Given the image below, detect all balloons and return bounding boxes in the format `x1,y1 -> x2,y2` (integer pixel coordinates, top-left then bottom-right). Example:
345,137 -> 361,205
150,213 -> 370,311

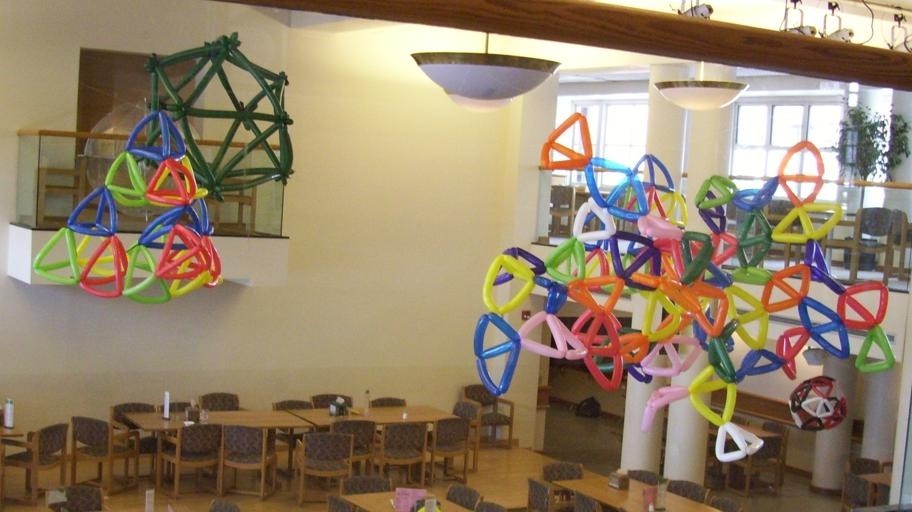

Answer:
31,32 -> 294,300
477,115 -> 897,463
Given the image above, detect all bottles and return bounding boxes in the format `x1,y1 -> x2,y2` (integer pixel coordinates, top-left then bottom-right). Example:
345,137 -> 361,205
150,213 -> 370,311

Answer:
363,389 -> 372,417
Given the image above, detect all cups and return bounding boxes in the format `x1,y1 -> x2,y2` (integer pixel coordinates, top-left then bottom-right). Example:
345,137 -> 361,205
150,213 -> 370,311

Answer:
425,497 -> 436,511
640,486 -> 666,512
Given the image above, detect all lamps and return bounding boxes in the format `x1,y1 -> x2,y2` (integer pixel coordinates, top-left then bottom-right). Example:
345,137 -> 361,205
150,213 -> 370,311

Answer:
778,0 -> 817,37
887,12 -> 912,53
820,0 -> 855,43
676,1 -> 713,19
410,30 -> 562,100
650,61 -> 749,111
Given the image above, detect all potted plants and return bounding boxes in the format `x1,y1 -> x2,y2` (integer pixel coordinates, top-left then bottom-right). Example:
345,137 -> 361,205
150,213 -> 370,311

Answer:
824,97 -> 911,271
